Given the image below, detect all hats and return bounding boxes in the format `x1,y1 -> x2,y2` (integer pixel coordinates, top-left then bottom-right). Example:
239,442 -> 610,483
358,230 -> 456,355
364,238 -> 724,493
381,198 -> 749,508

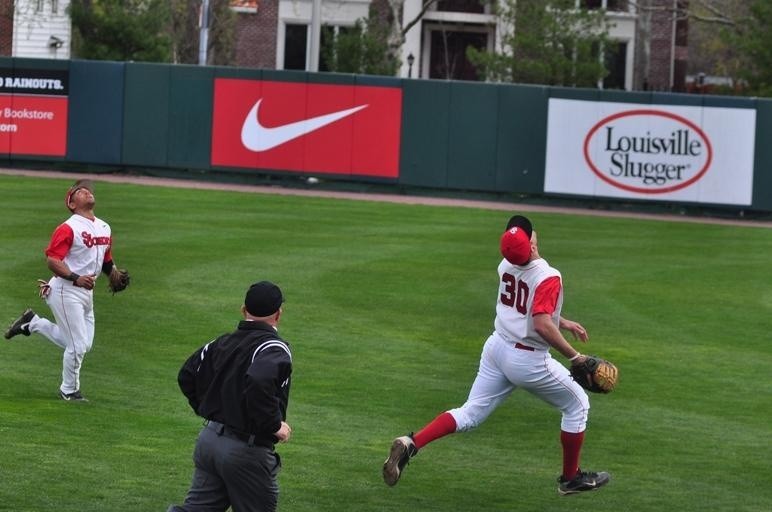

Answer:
245,280 -> 286,317
500,215 -> 532,265
65,179 -> 96,213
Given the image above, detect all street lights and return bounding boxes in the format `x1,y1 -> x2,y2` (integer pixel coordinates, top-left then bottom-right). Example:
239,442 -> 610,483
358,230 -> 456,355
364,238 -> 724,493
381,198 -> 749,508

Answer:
408,54 -> 415,78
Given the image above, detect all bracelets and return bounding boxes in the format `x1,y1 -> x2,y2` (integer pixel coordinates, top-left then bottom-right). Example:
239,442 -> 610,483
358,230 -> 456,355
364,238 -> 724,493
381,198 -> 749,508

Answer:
67,272 -> 80,281
567,352 -> 582,362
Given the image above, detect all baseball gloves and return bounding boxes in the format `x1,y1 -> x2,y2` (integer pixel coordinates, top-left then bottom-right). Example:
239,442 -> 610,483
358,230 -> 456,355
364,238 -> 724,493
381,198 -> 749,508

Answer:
110,267 -> 128,291
570,354 -> 618,393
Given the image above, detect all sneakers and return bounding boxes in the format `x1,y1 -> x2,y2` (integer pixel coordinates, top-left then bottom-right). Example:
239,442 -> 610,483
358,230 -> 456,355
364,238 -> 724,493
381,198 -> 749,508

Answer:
557,470 -> 610,495
382,435 -> 416,487
4,308 -> 35,340
59,390 -> 87,401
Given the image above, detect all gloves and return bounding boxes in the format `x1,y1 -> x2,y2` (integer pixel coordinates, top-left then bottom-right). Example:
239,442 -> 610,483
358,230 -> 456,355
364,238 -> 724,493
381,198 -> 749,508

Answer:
35,279 -> 51,300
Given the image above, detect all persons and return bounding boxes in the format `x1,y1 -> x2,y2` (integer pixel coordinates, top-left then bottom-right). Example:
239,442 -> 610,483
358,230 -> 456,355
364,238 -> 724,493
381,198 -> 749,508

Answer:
5,178 -> 130,402
383,214 -> 618,495
166,280 -> 293,511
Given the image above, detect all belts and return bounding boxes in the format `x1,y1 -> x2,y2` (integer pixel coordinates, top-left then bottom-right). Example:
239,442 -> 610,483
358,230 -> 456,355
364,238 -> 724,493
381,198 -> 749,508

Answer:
208,421 -> 274,451
